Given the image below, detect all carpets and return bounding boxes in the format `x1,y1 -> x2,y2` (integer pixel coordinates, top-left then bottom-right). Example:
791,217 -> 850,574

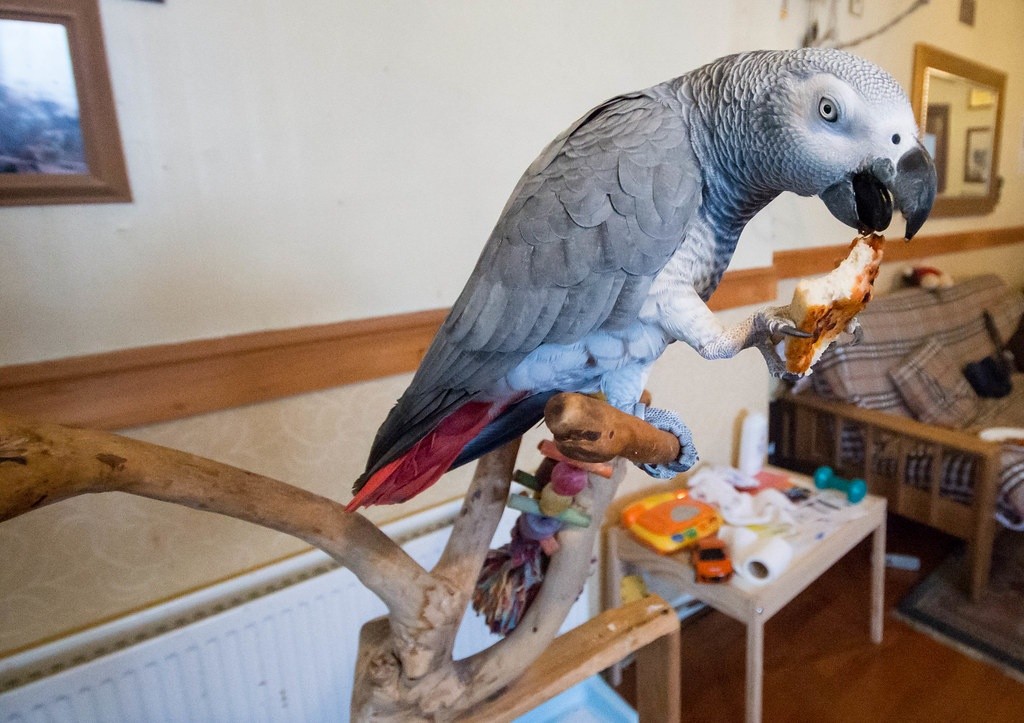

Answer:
892,536 -> 1024,684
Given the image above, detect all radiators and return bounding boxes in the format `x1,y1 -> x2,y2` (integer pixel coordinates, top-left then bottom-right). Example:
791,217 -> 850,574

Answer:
122,484 -> 590,723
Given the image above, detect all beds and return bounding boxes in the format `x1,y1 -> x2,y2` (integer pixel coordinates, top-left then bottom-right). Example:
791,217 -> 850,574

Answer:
794,392 -> 1024,604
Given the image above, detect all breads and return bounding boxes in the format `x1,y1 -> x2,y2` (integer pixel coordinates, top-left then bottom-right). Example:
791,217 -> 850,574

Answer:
785,234 -> 885,373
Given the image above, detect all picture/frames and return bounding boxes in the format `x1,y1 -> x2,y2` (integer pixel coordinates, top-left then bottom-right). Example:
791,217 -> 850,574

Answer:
926,102 -> 950,194
0,0 -> 133,205
968,87 -> 993,110
964,128 -> 992,184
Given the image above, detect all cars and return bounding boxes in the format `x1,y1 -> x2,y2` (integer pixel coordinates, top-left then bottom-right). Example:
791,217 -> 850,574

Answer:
690,538 -> 733,583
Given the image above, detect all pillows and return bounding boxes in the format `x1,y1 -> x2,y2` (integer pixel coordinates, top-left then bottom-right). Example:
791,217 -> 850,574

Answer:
889,337 -> 983,434
812,272 -> 1010,420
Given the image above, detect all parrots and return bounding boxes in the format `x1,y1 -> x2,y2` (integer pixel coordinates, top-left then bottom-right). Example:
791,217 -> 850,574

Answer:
333,48 -> 938,517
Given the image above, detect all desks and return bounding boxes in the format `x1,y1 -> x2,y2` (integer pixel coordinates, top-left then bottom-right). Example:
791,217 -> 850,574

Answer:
604,467 -> 887,723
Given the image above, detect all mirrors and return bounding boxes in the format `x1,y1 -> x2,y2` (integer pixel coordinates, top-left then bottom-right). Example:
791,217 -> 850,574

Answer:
911,43 -> 1007,219
